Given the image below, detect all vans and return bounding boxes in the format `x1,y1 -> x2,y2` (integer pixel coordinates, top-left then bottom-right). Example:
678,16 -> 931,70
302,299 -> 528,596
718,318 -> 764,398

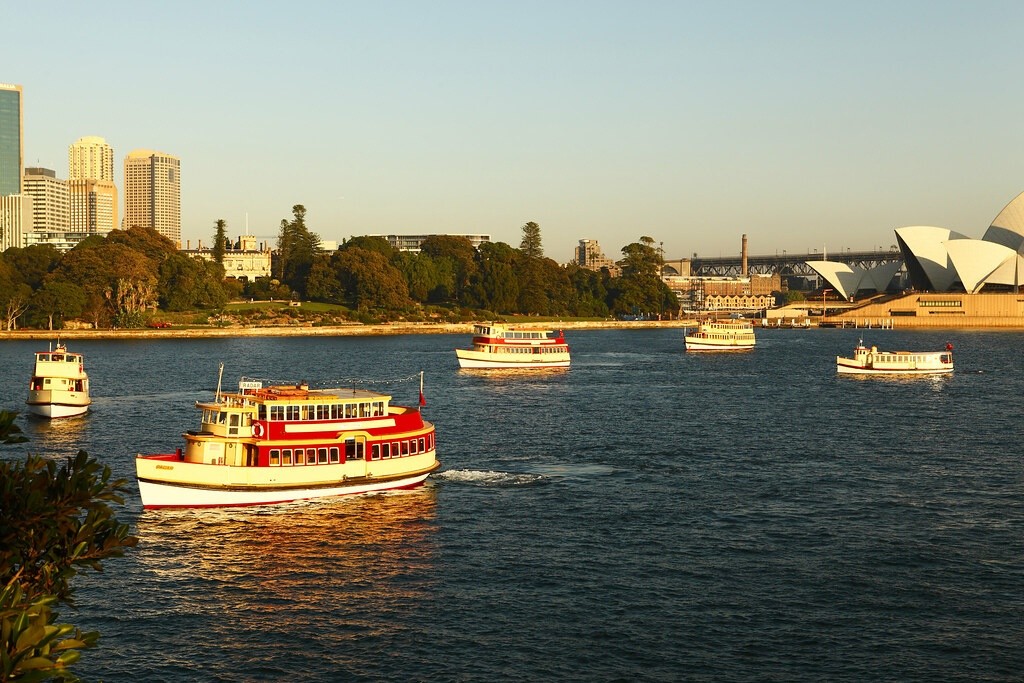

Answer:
732,313 -> 745,319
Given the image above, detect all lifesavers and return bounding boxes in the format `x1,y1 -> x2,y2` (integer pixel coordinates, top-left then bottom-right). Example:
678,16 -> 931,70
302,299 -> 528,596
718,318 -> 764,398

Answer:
251,423 -> 264,437
496,335 -> 501,340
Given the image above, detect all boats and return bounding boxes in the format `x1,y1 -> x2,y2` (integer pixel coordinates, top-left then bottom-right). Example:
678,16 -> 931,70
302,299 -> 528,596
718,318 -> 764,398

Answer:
454,317 -> 572,369
837,330 -> 955,375
136,360 -> 444,511
23,334 -> 92,424
683,305 -> 756,352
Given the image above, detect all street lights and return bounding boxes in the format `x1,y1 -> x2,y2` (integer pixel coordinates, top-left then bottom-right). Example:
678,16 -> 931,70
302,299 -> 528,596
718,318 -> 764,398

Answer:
823,289 -> 833,317
